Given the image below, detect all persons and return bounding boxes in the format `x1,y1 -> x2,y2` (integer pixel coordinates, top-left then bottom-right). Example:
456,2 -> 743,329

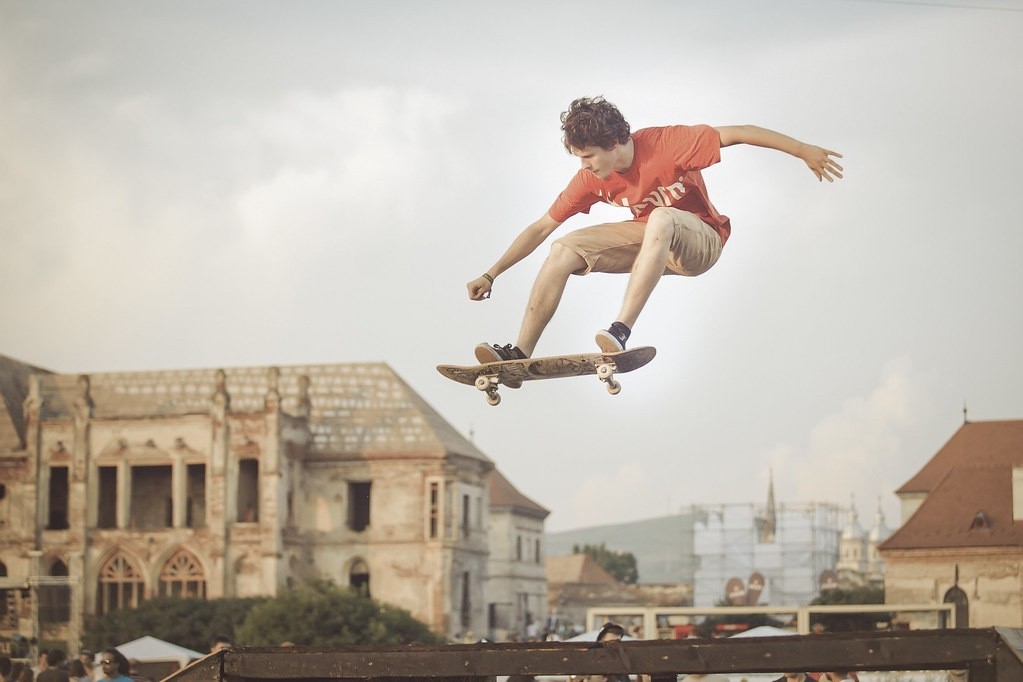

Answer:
0,657 -> 34,682
79,653 -> 96,682
467,95 -> 844,389
117,659 -> 145,682
211,637 -> 231,654
98,650 -> 135,682
31,650 -> 50,682
68,660 -> 91,682
770,671 -> 859,682
36,649 -> 69,682
573,622 -> 632,682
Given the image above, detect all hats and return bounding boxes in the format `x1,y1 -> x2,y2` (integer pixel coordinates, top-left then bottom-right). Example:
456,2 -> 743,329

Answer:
79,649 -> 95,662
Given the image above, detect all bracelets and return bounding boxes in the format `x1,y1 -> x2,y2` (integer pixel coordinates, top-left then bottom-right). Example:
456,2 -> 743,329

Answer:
483,273 -> 493,298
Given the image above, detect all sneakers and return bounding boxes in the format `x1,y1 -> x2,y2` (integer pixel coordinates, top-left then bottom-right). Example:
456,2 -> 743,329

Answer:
595,323 -> 627,353
474,342 -> 528,388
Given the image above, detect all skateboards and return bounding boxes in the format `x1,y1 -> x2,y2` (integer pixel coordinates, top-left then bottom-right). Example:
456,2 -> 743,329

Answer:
437,346 -> 658,405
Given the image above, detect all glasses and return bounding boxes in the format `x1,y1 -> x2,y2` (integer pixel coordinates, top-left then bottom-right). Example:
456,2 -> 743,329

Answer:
100,659 -> 117,664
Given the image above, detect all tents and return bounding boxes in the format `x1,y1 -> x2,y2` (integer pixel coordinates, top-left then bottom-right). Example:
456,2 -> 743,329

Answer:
95,635 -> 208,669
